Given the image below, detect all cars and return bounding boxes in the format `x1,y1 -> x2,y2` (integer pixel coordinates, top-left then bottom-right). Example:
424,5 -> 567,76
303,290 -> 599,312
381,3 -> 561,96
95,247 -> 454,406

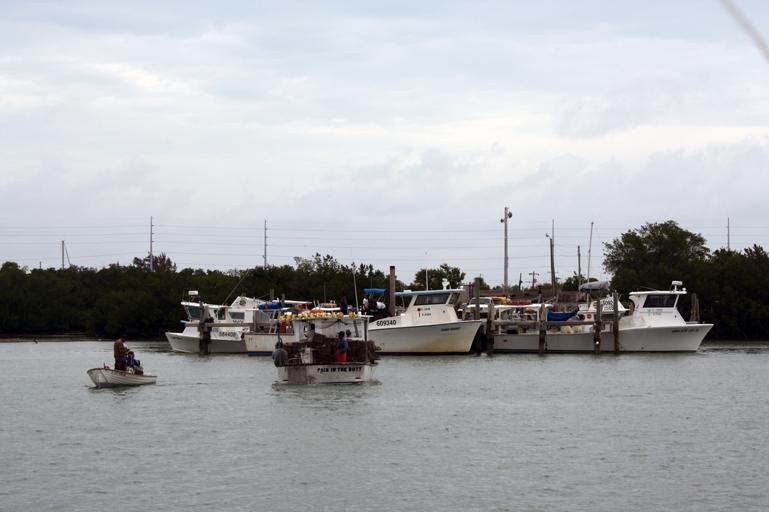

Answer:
464,296 -> 494,317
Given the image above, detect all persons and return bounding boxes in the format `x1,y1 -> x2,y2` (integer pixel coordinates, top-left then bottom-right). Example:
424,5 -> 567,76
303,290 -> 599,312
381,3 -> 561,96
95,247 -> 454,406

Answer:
376,296 -> 386,310
113,333 -> 127,372
272,341 -> 289,368
296,341 -> 320,365
304,323 -> 316,343
362,294 -> 369,315
334,330 -> 349,362
126,349 -> 145,375
340,294 -> 348,315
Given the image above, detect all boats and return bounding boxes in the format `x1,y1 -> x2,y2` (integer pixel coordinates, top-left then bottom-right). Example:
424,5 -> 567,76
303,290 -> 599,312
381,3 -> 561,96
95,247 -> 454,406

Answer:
271,305 -> 380,386
169,291 -> 310,354
85,361 -> 159,388
367,288 -> 487,355
492,283 -> 715,356
